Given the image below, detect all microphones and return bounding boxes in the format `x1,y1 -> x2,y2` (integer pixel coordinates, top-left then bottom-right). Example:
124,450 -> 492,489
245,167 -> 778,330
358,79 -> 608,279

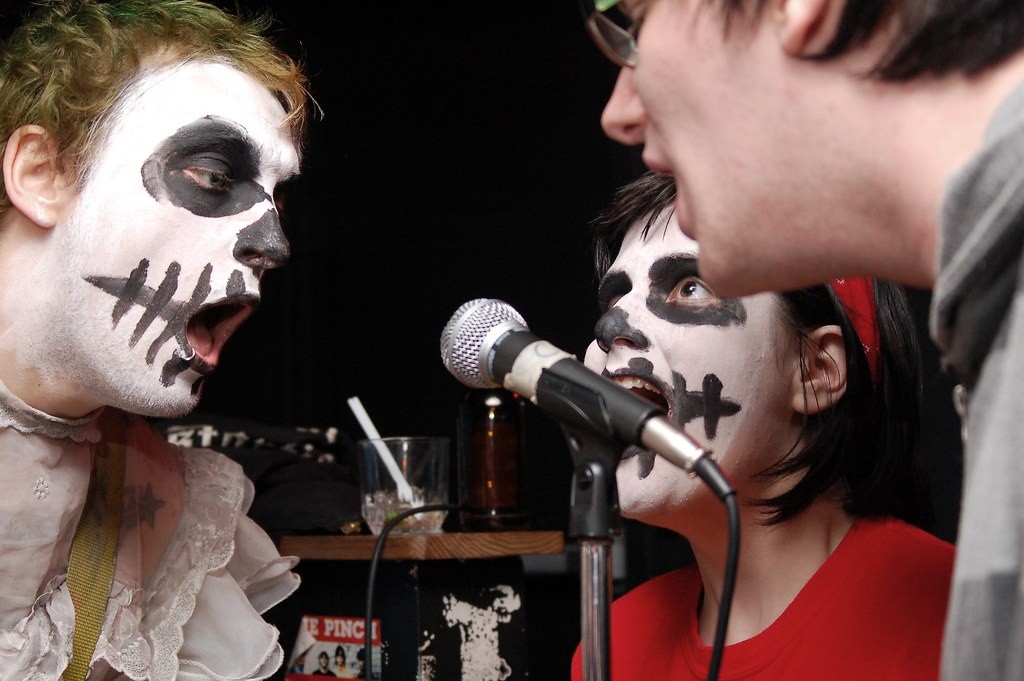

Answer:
441,298 -> 737,502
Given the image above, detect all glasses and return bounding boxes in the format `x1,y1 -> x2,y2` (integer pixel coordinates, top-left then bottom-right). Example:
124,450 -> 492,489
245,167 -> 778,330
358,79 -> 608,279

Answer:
585,0 -> 654,69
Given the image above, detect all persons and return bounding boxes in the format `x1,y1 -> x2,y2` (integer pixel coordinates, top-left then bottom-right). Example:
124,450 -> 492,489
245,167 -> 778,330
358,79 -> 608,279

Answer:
0,0 -> 326,681
571,168 -> 958,681
587,0 -> 1024,680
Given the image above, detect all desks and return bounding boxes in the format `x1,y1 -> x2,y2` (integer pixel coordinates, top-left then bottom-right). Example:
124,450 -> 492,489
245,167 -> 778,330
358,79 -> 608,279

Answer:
272,531 -> 566,681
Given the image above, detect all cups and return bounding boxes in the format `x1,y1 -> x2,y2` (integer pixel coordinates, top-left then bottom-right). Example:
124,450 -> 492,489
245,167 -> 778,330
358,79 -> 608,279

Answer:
355,435 -> 450,537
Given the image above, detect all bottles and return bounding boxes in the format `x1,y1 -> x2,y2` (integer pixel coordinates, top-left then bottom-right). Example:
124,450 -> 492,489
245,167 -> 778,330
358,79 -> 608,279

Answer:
457,384 -> 525,531
